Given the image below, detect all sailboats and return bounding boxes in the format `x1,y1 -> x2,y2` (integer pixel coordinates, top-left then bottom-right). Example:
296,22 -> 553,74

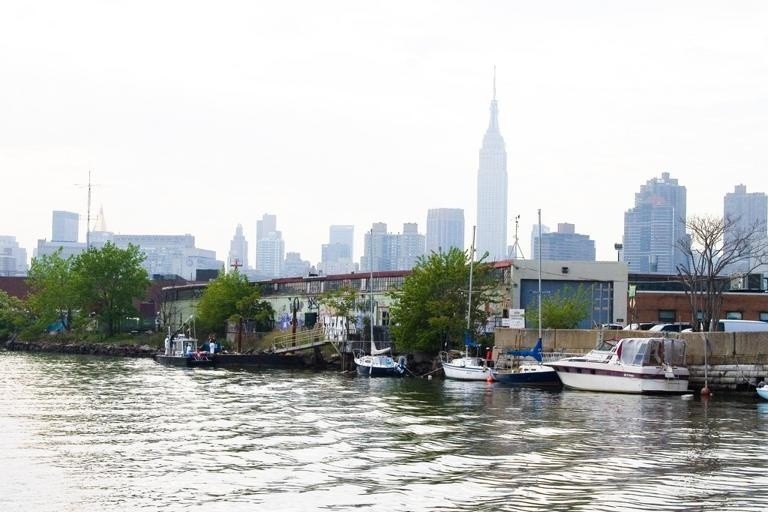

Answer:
491,207 -> 564,387
440,225 -> 506,382
353,228 -> 403,376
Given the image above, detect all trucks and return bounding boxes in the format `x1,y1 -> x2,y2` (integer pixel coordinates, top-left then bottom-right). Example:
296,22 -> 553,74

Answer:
623,317 -> 767,331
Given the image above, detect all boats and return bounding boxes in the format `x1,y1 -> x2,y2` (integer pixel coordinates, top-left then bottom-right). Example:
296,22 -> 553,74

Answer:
153,346 -> 308,371
540,337 -> 695,395
754,386 -> 768,402
